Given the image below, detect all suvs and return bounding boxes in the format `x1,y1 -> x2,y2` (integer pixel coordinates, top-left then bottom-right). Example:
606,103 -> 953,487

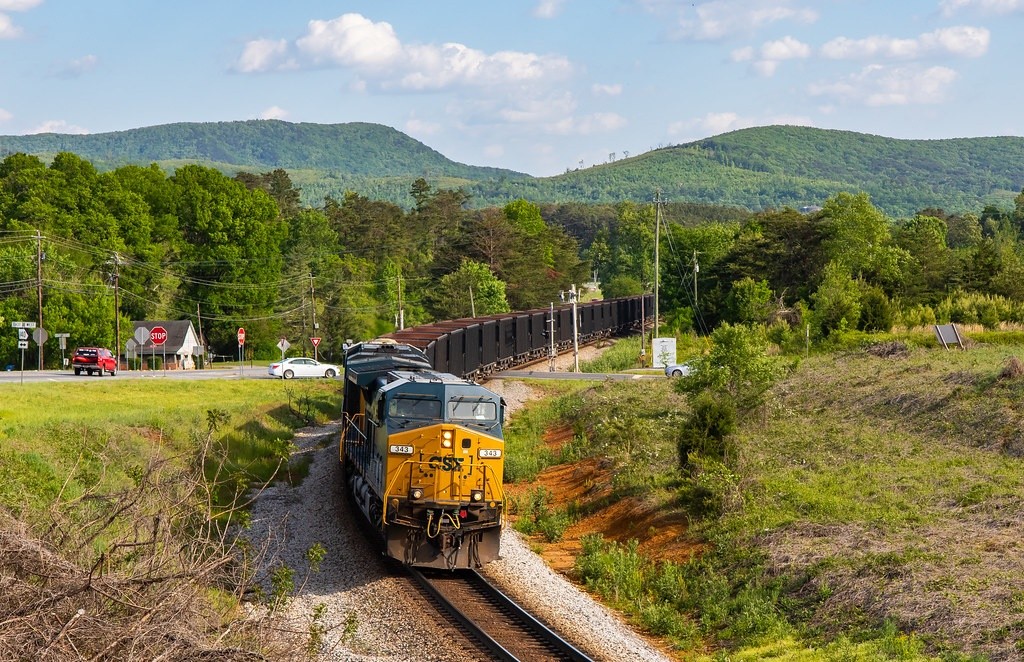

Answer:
72,347 -> 117,377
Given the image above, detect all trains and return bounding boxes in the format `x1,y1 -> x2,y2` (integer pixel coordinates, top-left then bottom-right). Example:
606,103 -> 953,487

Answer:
339,294 -> 655,572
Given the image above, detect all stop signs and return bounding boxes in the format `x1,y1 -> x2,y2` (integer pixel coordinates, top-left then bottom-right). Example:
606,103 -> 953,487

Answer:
237,328 -> 245,345
149,327 -> 167,345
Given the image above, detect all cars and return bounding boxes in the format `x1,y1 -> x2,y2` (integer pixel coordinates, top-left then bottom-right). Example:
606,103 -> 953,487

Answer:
269,357 -> 341,379
665,360 -> 724,379
